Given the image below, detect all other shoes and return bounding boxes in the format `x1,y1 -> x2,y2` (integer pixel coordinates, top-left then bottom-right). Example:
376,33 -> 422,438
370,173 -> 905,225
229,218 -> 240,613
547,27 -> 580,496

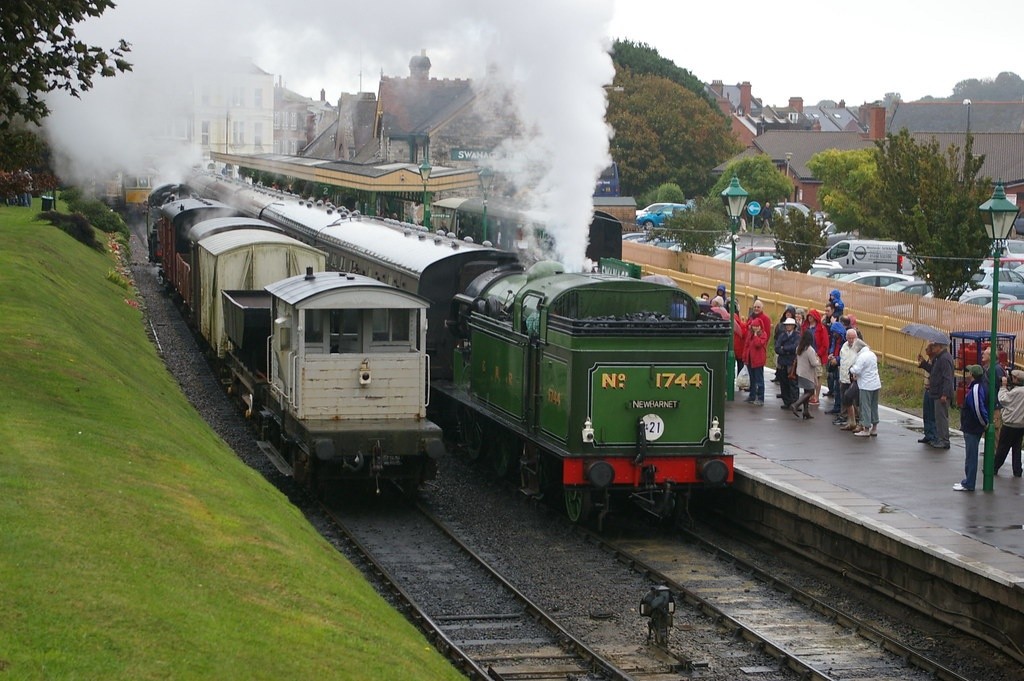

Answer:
1013,468 -> 1023,477
952,483 -> 968,491
870,431 -> 878,436
771,377 -> 780,385
824,410 -> 841,415
832,417 -> 848,426
839,424 -> 858,430
930,442 -> 951,449
854,431 -> 871,437
852,425 -> 865,433
917,437 -> 933,444
780,404 -> 801,411
982,468 -> 998,476
743,398 -> 764,406
822,392 -> 833,398
809,397 -> 820,405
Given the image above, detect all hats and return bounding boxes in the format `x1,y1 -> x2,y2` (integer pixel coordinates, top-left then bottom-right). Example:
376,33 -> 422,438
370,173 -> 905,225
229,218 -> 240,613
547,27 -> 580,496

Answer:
782,317 -> 796,325
966,364 -> 984,375
1011,370 -> 1024,379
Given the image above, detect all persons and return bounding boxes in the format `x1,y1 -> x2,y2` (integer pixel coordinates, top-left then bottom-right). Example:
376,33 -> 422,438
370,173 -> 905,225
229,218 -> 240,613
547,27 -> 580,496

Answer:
458,221 -> 465,240
0,170 -> 32,206
761,203 -> 772,234
981,347 -> 1006,454
738,209 -> 748,234
917,343 -> 954,448
392,213 -> 399,220
271,182 -> 333,202
770,290 -> 881,436
994,370 -> 1024,477
952,365 -> 989,491
438,221 -> 448,235
701,285 -> 771,404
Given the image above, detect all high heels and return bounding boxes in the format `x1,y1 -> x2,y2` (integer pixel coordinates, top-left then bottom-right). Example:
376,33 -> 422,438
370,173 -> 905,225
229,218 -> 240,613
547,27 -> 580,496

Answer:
802,411 -> 815,420
789,403 -> 800,418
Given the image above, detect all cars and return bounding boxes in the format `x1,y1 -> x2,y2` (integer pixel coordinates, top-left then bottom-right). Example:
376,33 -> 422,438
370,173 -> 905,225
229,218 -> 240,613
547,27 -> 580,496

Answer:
635,202 -> 1024,315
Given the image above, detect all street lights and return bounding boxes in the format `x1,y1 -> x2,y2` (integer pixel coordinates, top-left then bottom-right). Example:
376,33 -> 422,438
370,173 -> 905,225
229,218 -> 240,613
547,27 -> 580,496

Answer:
417,157 -> 433,230
978,176 -> 1022,494
720,172 -> 749,403
963,98 -> 972,150
478,166 -> 495,244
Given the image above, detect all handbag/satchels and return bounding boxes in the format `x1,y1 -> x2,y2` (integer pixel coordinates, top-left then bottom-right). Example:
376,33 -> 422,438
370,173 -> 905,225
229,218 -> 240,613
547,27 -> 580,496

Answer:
842,380 -> 859,407
788,356 -> 798,380
736,364 -> 751,389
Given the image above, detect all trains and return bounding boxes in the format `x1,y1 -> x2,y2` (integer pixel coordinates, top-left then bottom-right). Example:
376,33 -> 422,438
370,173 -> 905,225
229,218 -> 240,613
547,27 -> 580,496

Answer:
95,160 -> 153,213
144,182 -> 445,492
165,145 -> 742,533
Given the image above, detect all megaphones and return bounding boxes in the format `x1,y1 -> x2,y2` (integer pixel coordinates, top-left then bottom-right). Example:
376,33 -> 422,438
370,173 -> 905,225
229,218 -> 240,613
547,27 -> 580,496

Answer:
732,234 -> 741,244
725,236 -> 731,244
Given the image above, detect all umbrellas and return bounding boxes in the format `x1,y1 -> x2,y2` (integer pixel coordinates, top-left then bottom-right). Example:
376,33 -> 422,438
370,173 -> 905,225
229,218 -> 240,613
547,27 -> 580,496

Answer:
900,324 -> 951,360
642,272 -> 679,288
